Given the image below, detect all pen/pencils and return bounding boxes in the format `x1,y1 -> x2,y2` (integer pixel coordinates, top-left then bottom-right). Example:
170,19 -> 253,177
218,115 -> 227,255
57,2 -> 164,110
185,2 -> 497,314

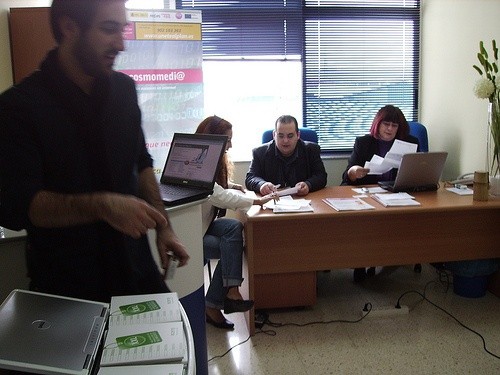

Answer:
273,191 -> 276,204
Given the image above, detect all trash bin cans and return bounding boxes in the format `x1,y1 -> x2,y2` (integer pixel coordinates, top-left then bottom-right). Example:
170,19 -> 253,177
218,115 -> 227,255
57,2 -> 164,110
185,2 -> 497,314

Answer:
454,259 -> 490,298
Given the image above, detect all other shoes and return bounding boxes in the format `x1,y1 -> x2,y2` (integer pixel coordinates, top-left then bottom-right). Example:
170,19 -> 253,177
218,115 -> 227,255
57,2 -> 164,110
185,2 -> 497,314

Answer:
353,274 -> 376,291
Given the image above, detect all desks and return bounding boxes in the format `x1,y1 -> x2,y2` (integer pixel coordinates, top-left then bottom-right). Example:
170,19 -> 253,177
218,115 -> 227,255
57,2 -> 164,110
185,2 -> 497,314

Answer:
225,181 -> 500,336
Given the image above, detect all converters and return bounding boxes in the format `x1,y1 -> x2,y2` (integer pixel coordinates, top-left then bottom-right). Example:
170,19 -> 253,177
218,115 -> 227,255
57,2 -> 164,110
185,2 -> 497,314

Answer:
254,314 -> 269,329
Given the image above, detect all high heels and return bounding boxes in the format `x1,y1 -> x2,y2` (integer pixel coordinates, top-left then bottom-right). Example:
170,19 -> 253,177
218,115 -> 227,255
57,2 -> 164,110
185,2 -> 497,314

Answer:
224,296 -> 255,313
205,310 -> 235,329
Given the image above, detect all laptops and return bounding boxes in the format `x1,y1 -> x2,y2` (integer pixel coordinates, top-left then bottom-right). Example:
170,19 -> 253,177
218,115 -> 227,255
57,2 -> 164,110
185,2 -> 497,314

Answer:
378,151 -> 449,194
0,289 -> 110,375
157,133 -> 229,207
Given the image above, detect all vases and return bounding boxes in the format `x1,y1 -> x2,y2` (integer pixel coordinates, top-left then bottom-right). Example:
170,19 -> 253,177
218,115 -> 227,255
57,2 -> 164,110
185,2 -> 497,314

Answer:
487,102 -> 500,197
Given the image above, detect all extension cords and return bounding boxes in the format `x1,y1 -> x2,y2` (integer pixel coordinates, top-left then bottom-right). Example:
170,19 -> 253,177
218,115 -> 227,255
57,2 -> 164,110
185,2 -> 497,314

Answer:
362,306 -> 409,319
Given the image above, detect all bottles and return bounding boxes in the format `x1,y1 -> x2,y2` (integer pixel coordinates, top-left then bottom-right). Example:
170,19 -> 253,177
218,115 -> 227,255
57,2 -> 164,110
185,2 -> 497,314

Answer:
473,171 -> 489,201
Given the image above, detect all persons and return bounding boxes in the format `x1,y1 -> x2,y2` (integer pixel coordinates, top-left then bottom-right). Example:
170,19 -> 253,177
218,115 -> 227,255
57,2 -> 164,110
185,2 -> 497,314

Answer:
197,116 -> 280,330
244,115 -> 329,196
0,0 -> 190,304
342,105 -> 421,290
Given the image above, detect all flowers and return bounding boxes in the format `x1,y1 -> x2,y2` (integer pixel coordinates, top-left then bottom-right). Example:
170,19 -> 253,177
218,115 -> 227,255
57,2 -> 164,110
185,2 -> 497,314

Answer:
472,40 -> 500,178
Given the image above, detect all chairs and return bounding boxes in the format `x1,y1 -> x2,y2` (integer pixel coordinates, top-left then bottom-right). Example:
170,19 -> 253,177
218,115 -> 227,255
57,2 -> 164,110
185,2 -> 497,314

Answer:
262,129 -> 318,145
406,121 -> 429,272
